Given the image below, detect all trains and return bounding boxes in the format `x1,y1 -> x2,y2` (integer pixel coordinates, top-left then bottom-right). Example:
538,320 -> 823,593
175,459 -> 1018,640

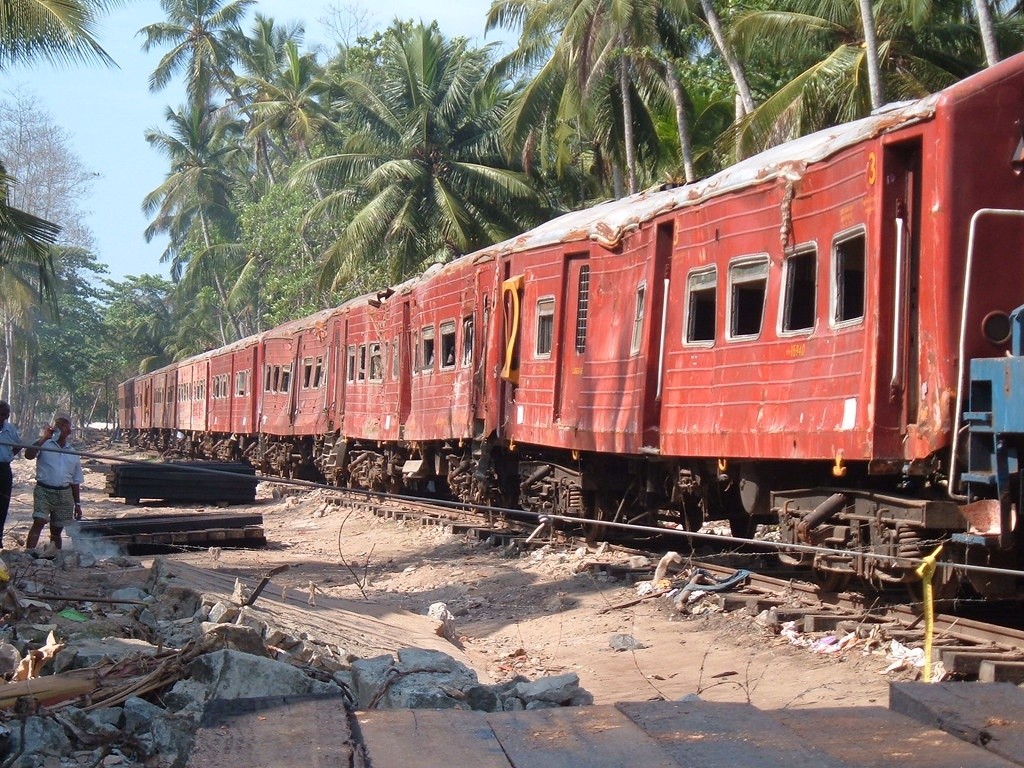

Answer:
116,50 -> 1024,624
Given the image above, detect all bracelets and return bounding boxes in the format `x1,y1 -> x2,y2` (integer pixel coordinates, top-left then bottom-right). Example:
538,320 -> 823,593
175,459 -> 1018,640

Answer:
75,502 -> 81,506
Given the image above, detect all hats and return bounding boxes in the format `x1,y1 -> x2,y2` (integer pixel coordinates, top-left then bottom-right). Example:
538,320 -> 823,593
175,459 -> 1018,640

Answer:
54,412 -> 72,422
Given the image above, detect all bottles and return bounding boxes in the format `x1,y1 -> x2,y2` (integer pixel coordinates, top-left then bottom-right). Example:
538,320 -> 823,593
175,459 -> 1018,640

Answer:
51,428 -> 61,443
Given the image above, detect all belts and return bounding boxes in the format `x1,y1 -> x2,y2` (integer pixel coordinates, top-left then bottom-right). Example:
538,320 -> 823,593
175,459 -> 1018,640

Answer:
37,481 -> 71,490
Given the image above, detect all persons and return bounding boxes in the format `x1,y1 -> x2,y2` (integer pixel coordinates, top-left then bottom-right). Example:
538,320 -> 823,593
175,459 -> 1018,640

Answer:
0,400 -> 23,548
24,412 -> 84,549
